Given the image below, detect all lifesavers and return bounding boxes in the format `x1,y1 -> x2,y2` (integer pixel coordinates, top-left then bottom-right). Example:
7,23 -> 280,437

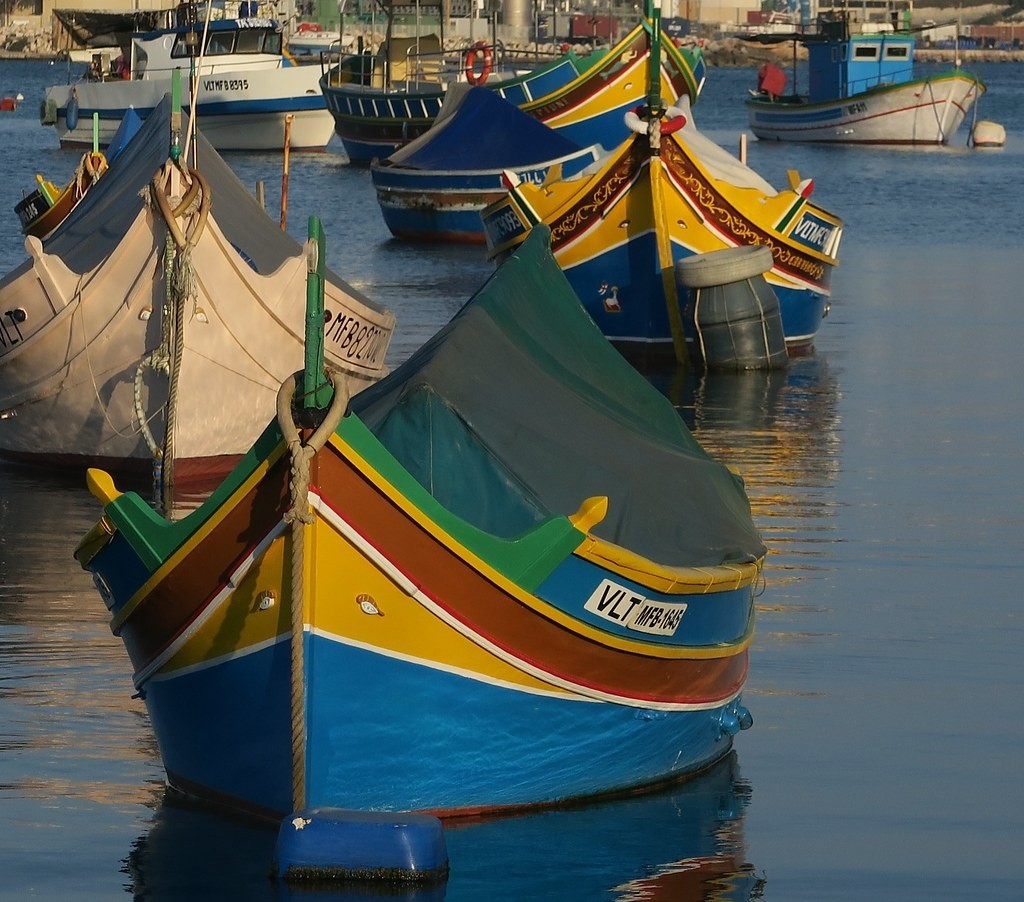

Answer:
624,104 -> 688,136
465,40 -> 493,85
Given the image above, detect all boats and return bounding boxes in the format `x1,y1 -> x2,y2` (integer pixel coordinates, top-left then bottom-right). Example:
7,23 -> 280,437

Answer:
1,67 -> 402,492
740,4 -> 990,146
475,1 -> 853,360
38,1 -> 335,155
71,210 -> 770,833
314,1 -> 710,170
367,75 -> 610,248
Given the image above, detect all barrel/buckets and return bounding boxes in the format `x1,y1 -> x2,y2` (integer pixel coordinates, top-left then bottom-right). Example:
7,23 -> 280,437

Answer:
682,273 -> 789,373
